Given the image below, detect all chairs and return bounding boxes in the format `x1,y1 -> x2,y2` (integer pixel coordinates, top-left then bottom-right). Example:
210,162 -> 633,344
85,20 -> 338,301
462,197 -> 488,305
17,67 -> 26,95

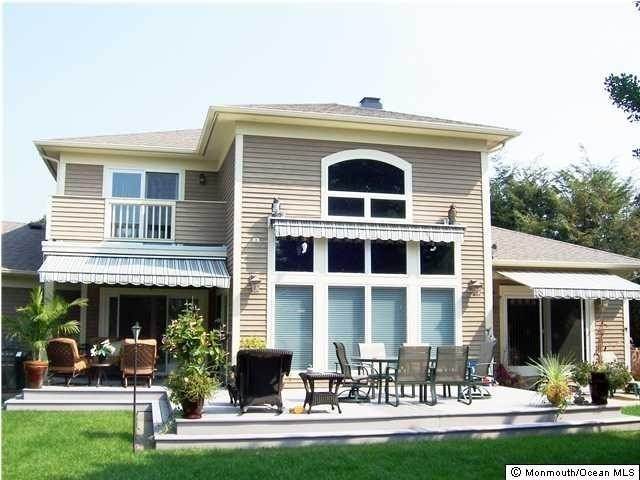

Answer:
226,349 -> 293,416
48,338 -> 94,386
120,338 -> 158,388
333,334 -> 495,407
600,350 -> 640,397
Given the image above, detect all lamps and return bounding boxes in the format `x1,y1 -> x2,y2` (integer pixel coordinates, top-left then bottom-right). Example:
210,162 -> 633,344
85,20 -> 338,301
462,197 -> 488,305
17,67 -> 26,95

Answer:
429,241 -> 437,252
467,279 -> 483,296
248,274 -> 262,293
301,237 -> 308,253
200,174 -> 206,183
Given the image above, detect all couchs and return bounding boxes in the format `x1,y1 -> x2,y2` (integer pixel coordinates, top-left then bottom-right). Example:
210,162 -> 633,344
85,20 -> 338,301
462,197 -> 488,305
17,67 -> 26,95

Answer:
86,337 -> 125,368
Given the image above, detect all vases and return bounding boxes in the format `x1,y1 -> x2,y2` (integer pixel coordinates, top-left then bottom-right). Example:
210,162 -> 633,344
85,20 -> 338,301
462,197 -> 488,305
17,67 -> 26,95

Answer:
98,355 -> 104,365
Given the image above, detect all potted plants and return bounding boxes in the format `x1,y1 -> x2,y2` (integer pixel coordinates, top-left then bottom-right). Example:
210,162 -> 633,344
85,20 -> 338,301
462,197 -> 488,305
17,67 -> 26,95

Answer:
164,367 -> 223,420
571,353 -> 633,405
523,352 -> 575,423
1,285 -> 90,389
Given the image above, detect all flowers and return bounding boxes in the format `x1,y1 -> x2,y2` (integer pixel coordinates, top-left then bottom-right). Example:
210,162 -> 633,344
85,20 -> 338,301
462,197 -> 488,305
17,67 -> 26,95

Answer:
90,338 -> 117,357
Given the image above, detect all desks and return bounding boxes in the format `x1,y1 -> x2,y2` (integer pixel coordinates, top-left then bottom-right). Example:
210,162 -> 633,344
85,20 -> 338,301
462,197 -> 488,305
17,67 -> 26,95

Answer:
299,372 -> 344,414
